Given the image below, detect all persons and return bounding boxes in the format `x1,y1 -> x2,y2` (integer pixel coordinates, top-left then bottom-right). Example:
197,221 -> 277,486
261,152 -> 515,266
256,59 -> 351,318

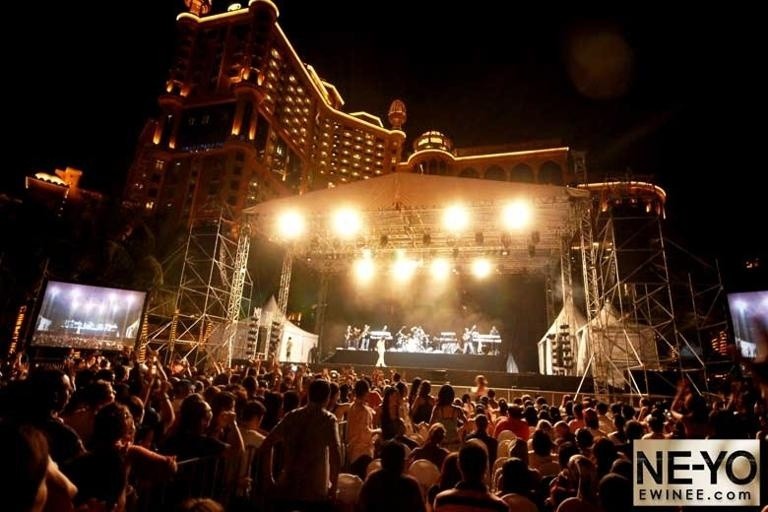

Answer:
1,323 -> 767,512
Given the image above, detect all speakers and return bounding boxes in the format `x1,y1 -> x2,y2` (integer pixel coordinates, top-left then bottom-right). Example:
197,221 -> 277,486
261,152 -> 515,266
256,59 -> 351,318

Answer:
514,375 -> 541,389
556,333 -> 564,366
430,370 -> 448,384
612,206 -> 651,281
201,222 -> 229,287
257,326 -> 267,354
